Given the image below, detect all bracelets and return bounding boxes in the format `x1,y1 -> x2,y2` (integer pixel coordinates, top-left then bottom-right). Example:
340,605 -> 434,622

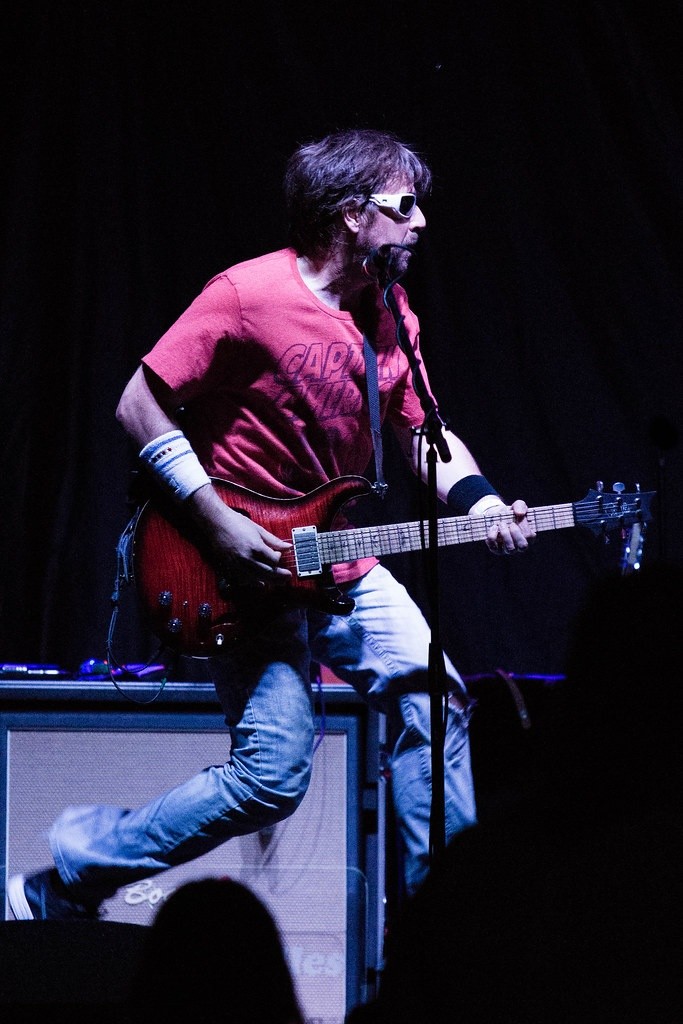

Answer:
446,474 -> 495,514
139,429 -> 212,500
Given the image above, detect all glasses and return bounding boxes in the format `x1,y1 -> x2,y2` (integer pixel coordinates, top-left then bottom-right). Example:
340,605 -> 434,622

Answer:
335,192 -> 418,219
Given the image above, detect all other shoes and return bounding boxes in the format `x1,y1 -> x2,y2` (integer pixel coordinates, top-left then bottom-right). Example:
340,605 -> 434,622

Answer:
6,867 -> 101,922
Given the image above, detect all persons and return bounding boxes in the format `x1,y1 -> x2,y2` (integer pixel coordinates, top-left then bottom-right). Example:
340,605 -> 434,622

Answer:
96,558 -> 682,1024
24,132 -> 533,924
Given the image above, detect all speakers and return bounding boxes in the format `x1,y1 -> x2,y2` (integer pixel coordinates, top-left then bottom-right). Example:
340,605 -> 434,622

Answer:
3,710 -> 364,1024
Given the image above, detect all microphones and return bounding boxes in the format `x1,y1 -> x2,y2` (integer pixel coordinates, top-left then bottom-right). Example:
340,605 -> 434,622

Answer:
361,244 -> 392,281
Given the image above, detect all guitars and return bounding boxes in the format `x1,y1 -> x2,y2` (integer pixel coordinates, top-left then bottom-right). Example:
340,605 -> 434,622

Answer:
107,471 -> 666,663
